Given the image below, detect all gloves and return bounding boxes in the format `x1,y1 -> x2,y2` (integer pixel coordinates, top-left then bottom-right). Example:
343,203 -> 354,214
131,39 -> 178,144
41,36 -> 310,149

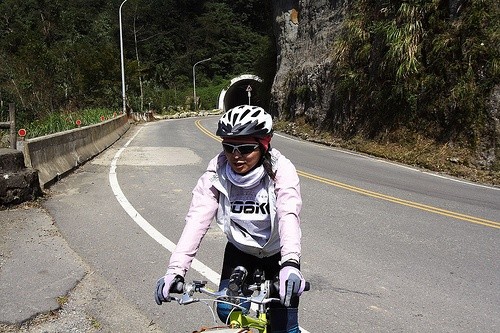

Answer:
154,275 -> 184,305
279,262 -> 305,307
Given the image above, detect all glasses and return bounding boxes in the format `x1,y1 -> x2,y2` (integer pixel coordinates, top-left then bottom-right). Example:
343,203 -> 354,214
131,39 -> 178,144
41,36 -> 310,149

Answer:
222,140 -> 260,154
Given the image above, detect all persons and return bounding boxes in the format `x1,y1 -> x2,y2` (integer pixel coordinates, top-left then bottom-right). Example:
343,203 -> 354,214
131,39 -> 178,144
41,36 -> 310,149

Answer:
153,104 -> 306,333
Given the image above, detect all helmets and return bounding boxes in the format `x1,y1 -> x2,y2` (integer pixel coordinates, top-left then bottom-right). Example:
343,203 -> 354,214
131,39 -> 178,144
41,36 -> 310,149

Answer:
216,105 -> 272,138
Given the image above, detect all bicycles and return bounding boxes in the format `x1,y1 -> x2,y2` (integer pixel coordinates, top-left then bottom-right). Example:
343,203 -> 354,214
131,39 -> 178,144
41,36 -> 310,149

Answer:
160,274 -> 310,333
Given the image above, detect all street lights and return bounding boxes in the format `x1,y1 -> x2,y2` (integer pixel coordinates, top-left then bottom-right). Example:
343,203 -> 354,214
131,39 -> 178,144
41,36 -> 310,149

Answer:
191,57 -> 211,116
